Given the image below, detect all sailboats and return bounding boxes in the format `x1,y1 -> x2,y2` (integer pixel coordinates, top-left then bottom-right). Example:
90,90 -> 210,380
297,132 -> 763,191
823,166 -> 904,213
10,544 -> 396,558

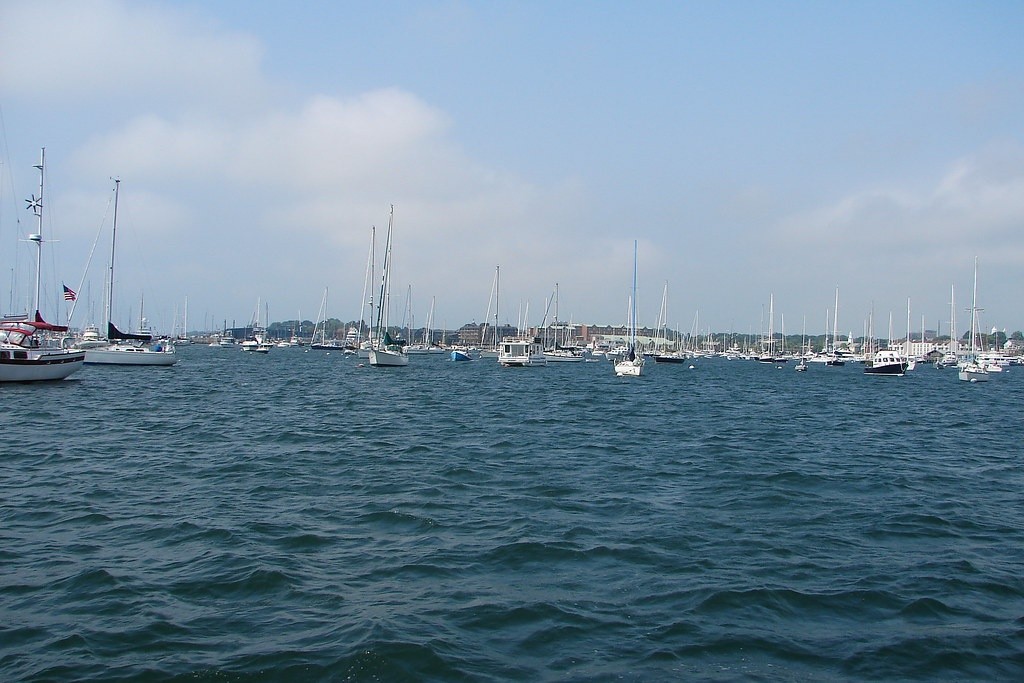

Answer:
0,127 -> 1024,383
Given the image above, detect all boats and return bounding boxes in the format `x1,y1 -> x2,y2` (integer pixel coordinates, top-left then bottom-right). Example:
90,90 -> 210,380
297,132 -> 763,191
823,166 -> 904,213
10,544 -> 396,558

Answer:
0,320 -> 86,382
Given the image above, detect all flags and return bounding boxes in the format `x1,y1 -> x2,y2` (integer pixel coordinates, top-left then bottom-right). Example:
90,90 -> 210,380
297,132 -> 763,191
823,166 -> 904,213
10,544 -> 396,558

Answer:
63,284 -> 77,302
